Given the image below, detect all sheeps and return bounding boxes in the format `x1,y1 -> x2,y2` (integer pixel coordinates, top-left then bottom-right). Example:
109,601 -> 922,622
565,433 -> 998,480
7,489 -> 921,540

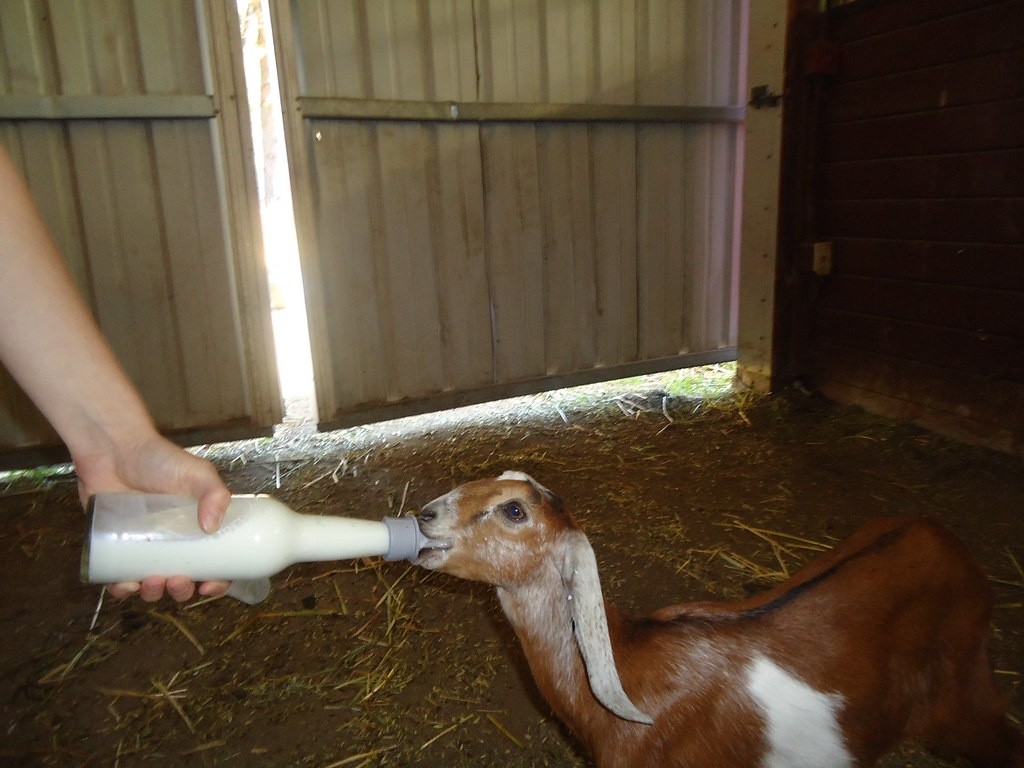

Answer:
407,469 -> 1024,768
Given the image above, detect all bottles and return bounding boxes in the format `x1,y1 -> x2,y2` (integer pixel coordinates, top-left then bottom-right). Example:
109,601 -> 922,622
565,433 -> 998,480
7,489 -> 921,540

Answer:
81,489 -> 454,587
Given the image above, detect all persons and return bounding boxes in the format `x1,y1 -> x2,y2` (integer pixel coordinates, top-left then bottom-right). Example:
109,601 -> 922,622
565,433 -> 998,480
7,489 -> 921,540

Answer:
0,150 -> 232,603
